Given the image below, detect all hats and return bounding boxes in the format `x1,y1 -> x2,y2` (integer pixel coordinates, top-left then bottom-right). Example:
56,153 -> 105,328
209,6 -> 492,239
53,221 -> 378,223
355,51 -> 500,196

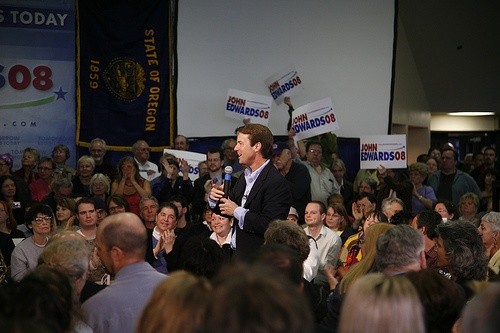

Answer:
272,142 -> 290,155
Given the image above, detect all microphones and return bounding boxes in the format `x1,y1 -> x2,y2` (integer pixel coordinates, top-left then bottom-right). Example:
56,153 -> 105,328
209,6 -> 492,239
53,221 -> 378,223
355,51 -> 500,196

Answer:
223,166 -> 233,199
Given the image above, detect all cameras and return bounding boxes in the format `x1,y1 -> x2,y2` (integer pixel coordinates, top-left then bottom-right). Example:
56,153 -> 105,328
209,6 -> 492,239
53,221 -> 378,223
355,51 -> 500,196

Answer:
12,202 -> 21,208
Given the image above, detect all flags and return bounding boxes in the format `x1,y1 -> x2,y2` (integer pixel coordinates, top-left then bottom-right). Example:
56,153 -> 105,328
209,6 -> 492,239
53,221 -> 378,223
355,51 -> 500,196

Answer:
74,0 -> 175,152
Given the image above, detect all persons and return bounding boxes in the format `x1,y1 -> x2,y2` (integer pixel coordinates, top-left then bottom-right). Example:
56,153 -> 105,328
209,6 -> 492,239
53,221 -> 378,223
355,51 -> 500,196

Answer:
0,135 -> 500,333
206,123 -> 292,253
286,97 -> 337,157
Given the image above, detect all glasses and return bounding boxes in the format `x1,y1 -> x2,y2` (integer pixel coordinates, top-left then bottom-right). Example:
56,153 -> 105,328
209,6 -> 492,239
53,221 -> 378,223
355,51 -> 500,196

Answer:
310,149 -> 321,153
35,216 -> 52,224
1,164 -> 8,167
38,167 -> 52,171
